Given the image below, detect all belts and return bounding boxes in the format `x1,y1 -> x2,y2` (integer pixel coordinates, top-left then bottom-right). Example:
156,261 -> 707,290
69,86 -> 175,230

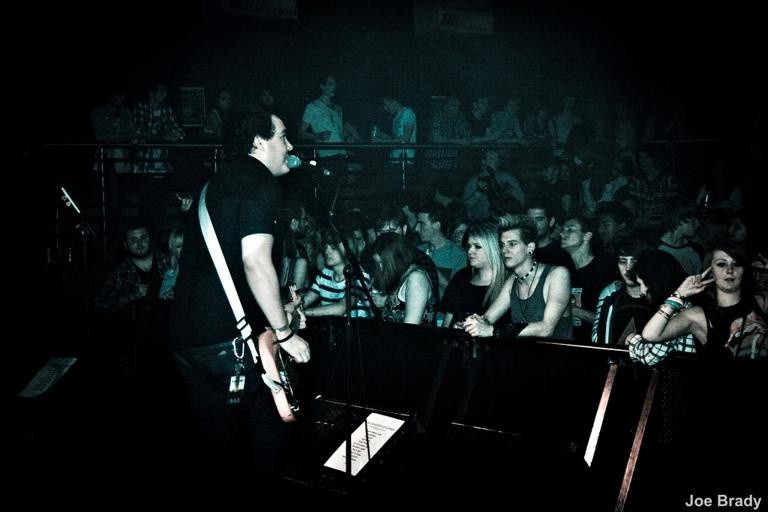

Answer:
191,345 -> 239,360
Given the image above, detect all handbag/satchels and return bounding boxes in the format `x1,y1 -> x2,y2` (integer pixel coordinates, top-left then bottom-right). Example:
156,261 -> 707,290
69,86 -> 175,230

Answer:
725,320 -> 768,358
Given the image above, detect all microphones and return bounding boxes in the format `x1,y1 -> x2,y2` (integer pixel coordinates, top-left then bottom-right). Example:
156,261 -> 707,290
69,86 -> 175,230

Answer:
287,155 -> 332,176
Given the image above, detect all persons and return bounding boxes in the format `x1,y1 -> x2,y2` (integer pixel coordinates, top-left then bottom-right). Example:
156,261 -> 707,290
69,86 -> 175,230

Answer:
100,75 -> 768,425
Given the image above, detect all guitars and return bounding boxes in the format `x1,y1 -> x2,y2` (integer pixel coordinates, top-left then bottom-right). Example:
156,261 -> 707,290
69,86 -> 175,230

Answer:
258,281 -> 299,424
